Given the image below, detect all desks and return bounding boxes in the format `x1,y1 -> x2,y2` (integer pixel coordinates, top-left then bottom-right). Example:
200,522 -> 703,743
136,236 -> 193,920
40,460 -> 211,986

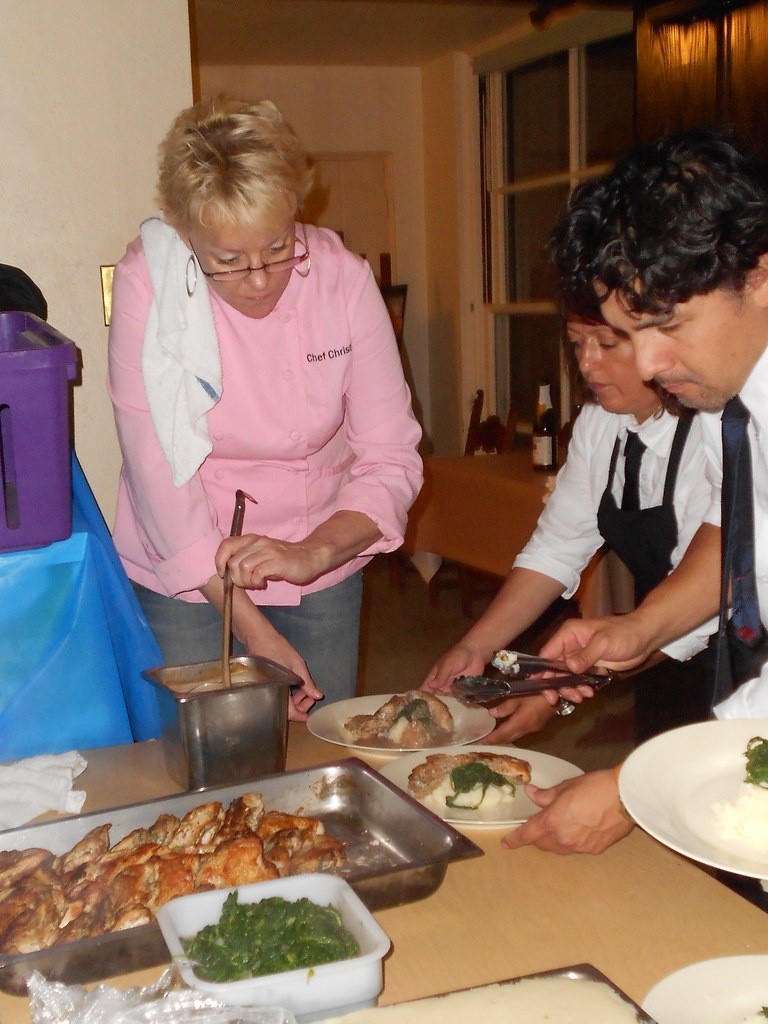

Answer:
0,453 -> 181,762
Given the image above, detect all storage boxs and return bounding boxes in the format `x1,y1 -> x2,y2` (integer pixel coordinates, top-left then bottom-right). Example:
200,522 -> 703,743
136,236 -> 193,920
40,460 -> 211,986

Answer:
0,312 -> 81,554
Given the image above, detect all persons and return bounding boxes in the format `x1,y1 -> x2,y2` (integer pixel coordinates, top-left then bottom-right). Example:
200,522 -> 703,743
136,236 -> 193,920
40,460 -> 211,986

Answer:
498,122 -> 767,912
110,100 -> 425,726
424,231 -> 734,750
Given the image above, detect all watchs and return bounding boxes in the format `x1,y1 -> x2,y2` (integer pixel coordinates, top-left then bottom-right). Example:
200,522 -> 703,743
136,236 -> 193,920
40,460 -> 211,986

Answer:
556,700 -> 576,715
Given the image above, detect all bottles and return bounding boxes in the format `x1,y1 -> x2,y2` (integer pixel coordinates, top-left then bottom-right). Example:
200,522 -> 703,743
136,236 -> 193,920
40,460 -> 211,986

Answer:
531,378 -> 557,474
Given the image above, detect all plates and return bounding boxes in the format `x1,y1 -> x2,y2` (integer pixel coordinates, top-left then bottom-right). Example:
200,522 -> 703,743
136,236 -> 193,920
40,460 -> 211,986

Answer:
641,952 -> 767,1024
617,717 -> 768,881
306,692 -> 496,760
379,745 -> 586,829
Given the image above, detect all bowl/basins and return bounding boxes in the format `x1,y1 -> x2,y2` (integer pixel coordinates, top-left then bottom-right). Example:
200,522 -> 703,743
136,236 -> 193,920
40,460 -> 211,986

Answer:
155,870 -> 392,1018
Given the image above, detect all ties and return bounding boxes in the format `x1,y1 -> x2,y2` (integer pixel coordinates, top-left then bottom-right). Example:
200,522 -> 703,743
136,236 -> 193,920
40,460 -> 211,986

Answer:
707,395 -> 765,719
619,428 -> 647,512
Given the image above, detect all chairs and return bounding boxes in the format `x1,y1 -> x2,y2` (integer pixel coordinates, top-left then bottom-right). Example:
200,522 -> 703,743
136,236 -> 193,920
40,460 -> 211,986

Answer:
427,391 -> 520,621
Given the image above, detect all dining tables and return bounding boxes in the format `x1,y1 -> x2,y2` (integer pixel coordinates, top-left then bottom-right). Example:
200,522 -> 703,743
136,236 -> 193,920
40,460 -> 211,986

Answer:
0,719 -> 768,1024
404,449 -> 634,619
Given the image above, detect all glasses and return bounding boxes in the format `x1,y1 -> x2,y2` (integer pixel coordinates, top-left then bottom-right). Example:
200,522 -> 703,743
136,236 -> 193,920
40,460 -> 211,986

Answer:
188,206 -> 310,282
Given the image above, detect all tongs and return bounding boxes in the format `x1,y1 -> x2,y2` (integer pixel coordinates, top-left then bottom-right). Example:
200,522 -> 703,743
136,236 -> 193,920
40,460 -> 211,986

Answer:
452,647 -> 613,704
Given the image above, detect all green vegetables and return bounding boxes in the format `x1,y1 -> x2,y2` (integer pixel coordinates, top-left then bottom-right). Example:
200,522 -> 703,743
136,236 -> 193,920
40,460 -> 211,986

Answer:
179,890 -> 360,982
397,698 -> 430,728
445,761 -> 515,810
741,736 -> 768,790
